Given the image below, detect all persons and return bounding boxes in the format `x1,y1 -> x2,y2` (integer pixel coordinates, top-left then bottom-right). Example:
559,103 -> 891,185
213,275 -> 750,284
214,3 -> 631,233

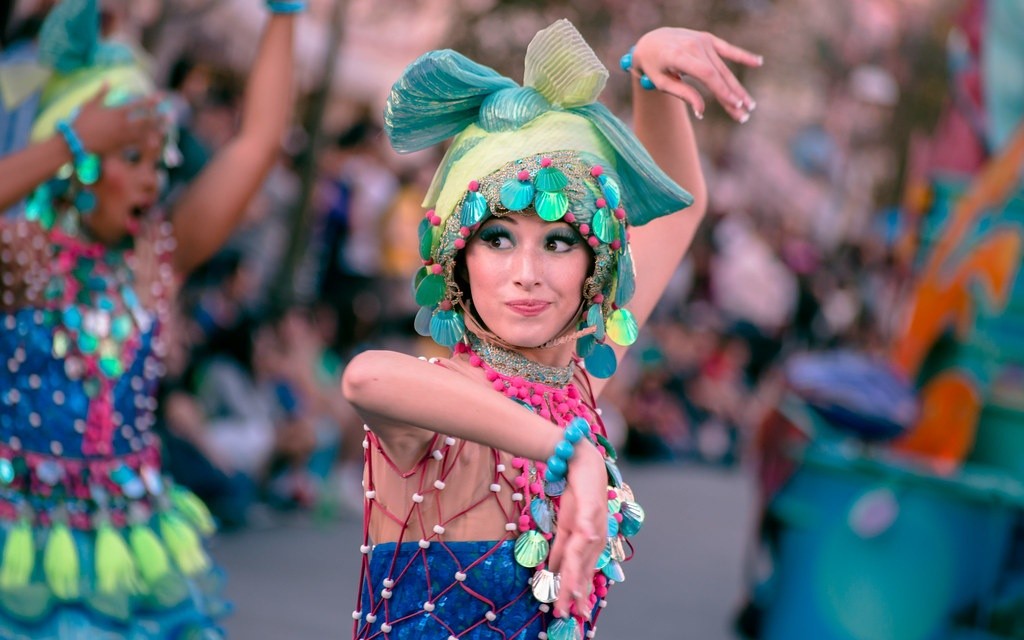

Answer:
0,1 -> 905,554
0,0 -> 307,640
336,15 -> 766,640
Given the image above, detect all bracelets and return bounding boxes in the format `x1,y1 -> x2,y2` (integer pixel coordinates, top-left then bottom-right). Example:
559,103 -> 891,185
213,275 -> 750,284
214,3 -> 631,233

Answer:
265,0 -> 306,14
545,415 -> 590,484
618,46 -> 656,91
54,120 -> 86,170
